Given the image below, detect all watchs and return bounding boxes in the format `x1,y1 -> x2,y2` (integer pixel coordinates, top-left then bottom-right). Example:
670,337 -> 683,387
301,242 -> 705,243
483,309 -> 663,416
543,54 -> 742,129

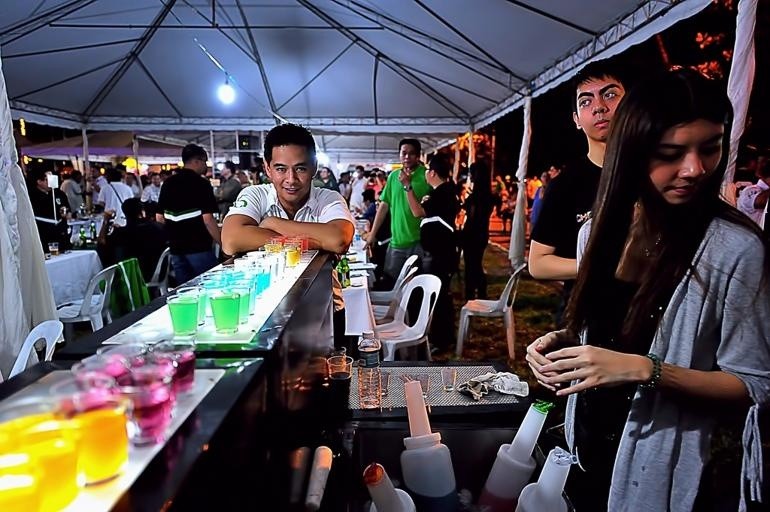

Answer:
403,184 -> 413,192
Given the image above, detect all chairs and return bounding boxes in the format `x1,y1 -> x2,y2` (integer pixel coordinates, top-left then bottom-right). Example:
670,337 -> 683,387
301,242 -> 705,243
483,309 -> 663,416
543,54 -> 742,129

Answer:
456,263 -> 526,361
144,247 -> 172,297
56,259 -> 151,333
8,320 -> 64,379
370,254 -> 418,323
371,267 -> 418,325
357,274 -> 442,361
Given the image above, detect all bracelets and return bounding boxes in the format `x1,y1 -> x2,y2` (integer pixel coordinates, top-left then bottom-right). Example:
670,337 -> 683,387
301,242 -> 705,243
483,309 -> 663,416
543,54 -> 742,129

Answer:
635,353 -> 662,392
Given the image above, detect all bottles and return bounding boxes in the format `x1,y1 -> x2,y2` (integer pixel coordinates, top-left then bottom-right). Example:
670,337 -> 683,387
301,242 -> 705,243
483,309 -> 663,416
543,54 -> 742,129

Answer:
400,381 -> 458,512
358,330 -> 381,410
477,402 -> 549,512
353,230 -> 362,254
362,463 -> 417,512
514,447 -> 573,512
90,221 -> 96,241
335,253 -> 350,288
78,224 -> 86,249
80,202 -> 87,217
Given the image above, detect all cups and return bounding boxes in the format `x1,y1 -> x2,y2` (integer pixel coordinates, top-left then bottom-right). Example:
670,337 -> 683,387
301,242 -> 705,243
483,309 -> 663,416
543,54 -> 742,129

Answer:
45,254 -> 50,259
414,374 -> 430,400
380,368 -> 391,397
48,242 -> 59,256
0,339 -> 196,511
440,368 -> 457,392
166,233 -> 310,335
326,356 -> 353,380
330,347 -> 346,373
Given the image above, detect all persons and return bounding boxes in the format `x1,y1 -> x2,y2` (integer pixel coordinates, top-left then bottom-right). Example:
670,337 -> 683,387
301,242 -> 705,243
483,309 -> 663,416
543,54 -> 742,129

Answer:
398,154 -> 459,356
524,66 -> 770,512
25,160 -> 183,279
219,123 -> 357,445
200,155 -> 268,228
154,141 -> 221,285
362,137 -> 431,292
312,164 -> 393,278
736,154 -> 770,236
527,58 -> 630,329
493,163 -> 567,239
460,161 -> 494,304
